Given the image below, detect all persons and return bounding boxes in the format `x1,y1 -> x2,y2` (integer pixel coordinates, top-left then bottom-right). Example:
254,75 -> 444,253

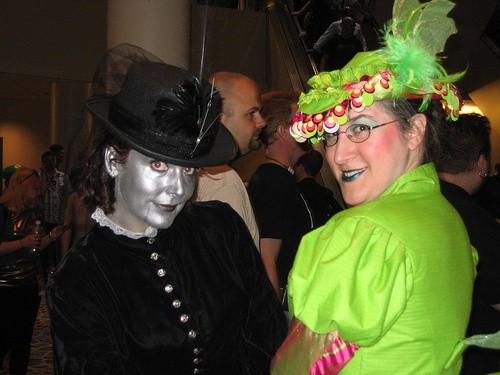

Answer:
0,0 -> 500,375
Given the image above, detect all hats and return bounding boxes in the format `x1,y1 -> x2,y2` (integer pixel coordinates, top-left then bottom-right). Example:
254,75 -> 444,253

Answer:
0,164 -> 25,182
84,43 -> 237,168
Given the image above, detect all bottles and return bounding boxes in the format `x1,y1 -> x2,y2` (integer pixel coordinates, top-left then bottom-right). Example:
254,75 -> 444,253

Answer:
30,220 -> 42,253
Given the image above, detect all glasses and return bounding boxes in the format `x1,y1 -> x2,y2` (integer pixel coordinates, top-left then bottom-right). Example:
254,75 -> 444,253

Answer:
320,118 -> 403,147
19,169 -> 39,185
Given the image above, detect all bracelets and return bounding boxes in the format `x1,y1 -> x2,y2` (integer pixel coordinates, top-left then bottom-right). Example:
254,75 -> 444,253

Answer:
48,233 -> 52,241
49,231 -> 56,240
20,239 -> 23,248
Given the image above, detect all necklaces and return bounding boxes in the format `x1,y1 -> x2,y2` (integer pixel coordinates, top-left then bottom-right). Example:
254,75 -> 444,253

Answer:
265,155 -> 294,175
